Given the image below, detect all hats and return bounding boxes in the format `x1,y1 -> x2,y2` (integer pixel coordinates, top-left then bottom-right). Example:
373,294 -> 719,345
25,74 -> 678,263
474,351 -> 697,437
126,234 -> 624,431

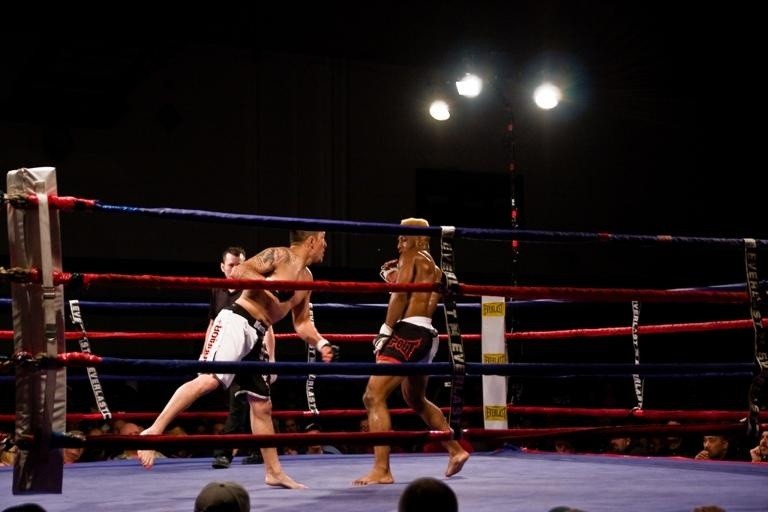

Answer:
194,482 -> 250,512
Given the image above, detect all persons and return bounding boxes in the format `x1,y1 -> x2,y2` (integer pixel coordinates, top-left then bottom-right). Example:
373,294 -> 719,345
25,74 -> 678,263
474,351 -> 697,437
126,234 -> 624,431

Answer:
352,218 -> 470,486
135,231 -> 336,490
207,248 -> 278,469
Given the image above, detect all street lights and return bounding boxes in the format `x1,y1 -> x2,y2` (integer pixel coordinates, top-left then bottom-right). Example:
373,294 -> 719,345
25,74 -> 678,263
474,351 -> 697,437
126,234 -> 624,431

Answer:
427,71 -> 563,284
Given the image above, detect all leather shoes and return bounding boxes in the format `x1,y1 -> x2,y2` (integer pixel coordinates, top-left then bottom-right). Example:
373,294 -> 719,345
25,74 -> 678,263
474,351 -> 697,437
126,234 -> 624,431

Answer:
242,449 -> 264,464
211,454 -> 232,469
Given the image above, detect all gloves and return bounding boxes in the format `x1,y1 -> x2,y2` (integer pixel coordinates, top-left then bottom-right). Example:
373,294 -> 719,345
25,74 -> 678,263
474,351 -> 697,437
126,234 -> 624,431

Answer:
379,258 -> 399,294
264,276 -> 295,303
372,322 -> 395,354
316,338 -> 341,363
